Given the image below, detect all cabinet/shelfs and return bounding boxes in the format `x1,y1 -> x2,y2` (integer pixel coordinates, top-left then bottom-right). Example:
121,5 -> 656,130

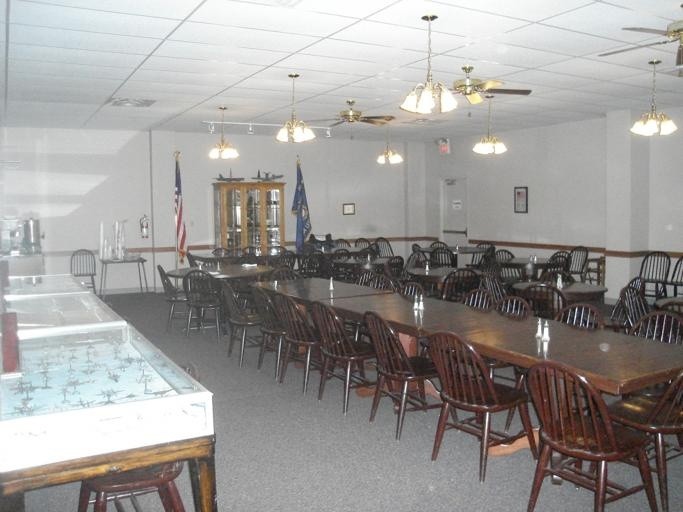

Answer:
220,186 -> 281,248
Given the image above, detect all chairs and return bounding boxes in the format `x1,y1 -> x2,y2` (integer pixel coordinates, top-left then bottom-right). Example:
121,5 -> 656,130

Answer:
364,310 -> 457,443
522,365 -> 657,512
271,294 -> 351,395
309,301 -> 394,413
155,238 -> 683,344
449,296 -> 530,382
70,249 -> 96,295
220,278 -> 282,367
506,304 -> 603,431
250,285 -> 311,381
621,311 -> 680,453
426,336 -> 539,482
416,291 -> 490,354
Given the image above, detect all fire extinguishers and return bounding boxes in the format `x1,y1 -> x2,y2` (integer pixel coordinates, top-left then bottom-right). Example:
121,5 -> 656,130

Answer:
139,214 -> 150,238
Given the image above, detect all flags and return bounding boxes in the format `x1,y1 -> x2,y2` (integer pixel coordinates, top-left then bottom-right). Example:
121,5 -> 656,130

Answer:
291,159 -> 312,251
174,159 -> 188,264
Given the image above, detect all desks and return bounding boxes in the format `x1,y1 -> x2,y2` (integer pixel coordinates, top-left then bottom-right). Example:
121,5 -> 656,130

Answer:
100,256 -> 148,301
0,273 -> 218,512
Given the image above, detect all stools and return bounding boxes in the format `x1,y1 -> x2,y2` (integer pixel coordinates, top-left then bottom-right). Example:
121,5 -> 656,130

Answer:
78,461 -> 186,512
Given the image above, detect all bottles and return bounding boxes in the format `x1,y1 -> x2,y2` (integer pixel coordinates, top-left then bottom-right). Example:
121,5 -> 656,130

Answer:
529,254 -> 537,262
455,244 -> 458,249
534,339 -> 548,361
329,276 -> 334,290
425,261 -> 429,271
412,292 -> 424,309
321,245 -> 324,252
533,319 -> 551,341
414,309 -> 423,324
198,263 -> 201,270
557,274 -> 561,285
367,254 -> 370,261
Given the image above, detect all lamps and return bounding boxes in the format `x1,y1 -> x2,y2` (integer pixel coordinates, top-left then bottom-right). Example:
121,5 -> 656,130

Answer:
400,15 -> 457,114
376,141 -> 402,164
276,74 -> 314,142
208,106 -> 238,160
473,95 -> 507,155
630,59 -> 677,138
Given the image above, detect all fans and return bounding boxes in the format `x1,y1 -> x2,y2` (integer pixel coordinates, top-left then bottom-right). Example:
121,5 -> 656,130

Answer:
599,20 -> 683,69
330,109 -> 394,128
438,65 -> 503,104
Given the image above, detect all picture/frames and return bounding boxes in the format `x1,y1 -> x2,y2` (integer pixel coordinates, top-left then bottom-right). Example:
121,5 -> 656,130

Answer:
343,203 -> 355,215
514,186 -> 528,213
